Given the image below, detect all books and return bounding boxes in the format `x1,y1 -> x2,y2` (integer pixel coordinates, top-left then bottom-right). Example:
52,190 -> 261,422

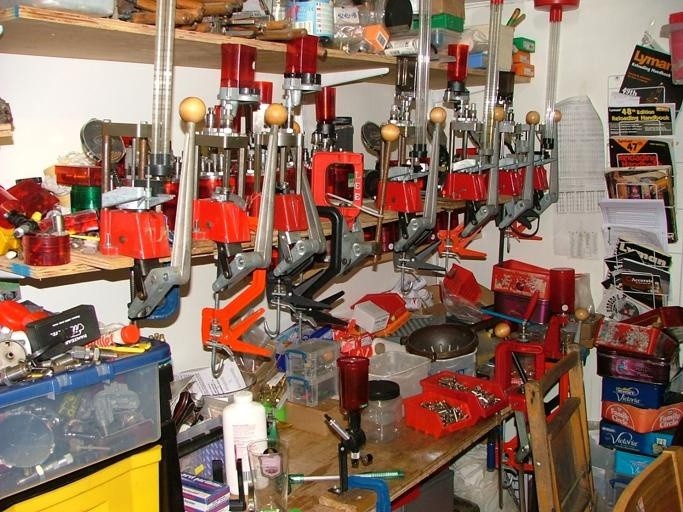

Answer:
596,45 -> 683,321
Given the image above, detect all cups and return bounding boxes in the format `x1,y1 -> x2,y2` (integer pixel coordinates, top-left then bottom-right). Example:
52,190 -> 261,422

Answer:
246,438 -> 290,511
336,356 -> 370,415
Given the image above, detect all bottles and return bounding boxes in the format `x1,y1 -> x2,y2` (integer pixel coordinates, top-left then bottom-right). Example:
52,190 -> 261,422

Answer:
222,390 -> 271,496
359,380 -> 403,444
269,0 -> 386,58
